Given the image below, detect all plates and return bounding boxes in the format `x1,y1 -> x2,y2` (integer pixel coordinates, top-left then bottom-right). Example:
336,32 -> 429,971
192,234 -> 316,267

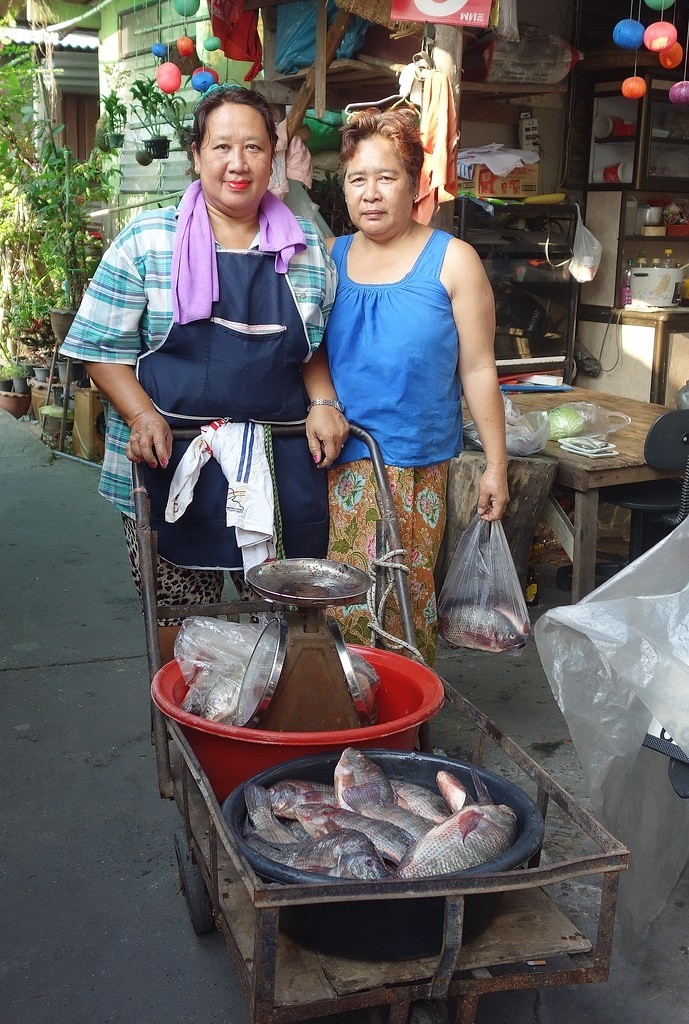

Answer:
558,437 -> 621,457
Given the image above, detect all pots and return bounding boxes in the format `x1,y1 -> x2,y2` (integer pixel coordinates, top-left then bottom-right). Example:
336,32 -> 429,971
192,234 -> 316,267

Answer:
630,268 -> 684,307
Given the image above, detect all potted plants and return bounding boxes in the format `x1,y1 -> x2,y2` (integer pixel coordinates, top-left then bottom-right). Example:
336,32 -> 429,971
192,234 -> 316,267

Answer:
0,41 -> 127,394
129,74 -> 186,159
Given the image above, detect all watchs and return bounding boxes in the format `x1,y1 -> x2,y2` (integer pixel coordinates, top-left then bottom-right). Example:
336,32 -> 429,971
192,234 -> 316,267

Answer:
307,398 -> 344,412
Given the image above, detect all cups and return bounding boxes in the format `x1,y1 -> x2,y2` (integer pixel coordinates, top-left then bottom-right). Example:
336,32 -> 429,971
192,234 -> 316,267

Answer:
592,115 -> 635,138
594,160 -> 632,184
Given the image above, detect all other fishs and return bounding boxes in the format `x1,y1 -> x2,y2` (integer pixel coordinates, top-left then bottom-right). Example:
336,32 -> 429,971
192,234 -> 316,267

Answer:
181,647 -> 380,726
437,590 -> 530,653
244,748 -> 519,881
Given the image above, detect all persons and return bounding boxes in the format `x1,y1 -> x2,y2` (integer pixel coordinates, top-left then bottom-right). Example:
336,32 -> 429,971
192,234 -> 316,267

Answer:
323,107 -> 513,668
58,87 -> 351,666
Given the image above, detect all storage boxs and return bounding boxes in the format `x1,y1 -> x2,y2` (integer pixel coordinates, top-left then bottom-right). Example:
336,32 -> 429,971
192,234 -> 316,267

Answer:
457,163 -> 540,197
662,225 -> 689,237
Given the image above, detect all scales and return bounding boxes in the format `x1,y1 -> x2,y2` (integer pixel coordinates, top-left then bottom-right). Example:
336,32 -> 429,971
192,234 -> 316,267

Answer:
234,556 -> 383,734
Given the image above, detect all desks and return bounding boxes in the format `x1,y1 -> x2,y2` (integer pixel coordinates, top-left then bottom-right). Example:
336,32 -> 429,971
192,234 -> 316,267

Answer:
460,383 -> 686,604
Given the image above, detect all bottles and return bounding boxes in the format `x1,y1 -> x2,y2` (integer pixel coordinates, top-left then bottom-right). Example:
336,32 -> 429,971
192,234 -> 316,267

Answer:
620,250 -> 689,309
626,197 -> 646,234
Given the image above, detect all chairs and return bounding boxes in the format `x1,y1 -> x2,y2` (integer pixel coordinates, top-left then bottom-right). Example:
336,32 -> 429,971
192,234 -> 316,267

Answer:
557,408 -> 689,592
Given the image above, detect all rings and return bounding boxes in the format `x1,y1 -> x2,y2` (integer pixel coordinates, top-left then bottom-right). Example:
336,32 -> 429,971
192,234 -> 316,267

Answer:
342,444 -> 344,448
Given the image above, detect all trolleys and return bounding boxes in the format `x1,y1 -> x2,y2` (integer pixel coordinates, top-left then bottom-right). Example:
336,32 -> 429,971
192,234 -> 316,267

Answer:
132,423 -> 630,1024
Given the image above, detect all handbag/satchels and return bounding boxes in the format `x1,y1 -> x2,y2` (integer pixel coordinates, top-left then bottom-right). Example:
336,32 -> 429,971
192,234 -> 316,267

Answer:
462,393 -> 549,456
569,204 -> 603,283
434,510 -> 531,653
549,403 -> 631,441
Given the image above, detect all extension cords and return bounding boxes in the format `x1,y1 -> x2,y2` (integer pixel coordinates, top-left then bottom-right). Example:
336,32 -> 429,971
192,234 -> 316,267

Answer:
518,112 -> 539,155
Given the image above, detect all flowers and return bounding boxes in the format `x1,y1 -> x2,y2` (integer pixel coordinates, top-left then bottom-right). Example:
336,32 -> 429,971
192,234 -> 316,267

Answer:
11,313 -> 57,356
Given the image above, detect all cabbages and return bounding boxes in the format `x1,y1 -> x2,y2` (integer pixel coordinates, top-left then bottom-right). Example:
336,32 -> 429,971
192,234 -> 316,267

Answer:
547,408 -> 585,440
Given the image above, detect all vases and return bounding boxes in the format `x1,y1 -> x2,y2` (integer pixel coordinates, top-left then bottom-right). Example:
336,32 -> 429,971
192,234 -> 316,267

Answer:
31,387 -> 55,421
56,361 -> 85,385
27,364 -> 40,377
39,411 -> 74,438
33,367 -> 50,382
0,391 -> 31,420
44,351 -> 58,367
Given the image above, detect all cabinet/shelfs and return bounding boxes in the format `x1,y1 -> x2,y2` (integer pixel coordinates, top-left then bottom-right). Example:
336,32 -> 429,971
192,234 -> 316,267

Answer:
578,66 -> 688,405
74,387 -> 111,462
455,198 -> 583,386
557,0 -> 689,409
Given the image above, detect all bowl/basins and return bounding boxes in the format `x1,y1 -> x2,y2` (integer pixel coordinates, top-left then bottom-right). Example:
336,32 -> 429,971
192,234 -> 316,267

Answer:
149,644 -> 445,809
645,206 -> 662,226
222,748 -> 545,960
666,224 -> 689,236
